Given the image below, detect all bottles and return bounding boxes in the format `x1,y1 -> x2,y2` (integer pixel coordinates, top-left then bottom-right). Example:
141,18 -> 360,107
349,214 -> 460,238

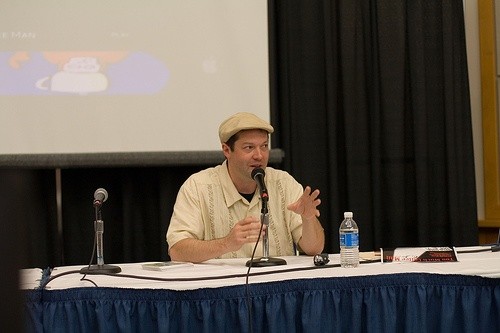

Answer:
339,212 -> 359,268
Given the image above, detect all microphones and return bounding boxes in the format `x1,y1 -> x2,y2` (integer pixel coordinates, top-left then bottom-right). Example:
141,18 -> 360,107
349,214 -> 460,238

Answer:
93,188 -> 108,208
251,167 -> 269,200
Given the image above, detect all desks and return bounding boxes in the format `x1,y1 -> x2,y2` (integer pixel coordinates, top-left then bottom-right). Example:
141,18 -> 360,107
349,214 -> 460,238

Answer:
17,246 -> 500,333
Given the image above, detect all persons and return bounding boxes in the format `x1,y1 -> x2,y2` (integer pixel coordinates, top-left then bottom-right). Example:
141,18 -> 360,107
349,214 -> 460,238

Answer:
165,112 -> 326,263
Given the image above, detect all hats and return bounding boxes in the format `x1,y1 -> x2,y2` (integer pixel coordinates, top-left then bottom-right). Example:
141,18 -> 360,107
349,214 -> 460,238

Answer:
218,111 -> 274,144
